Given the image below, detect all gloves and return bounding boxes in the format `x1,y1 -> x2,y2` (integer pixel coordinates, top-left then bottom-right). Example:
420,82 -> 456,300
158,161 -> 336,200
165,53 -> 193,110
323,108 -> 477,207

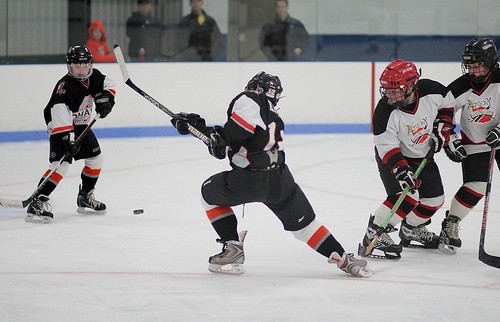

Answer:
429,122 -> 446,153
170,112 -> 200,135
94,93 -> 112,118
485,127 -> 500,147
442,132 -> 467,162
209,131 -> 226,159
61,134 -> 81,156
393,165 -> 422,195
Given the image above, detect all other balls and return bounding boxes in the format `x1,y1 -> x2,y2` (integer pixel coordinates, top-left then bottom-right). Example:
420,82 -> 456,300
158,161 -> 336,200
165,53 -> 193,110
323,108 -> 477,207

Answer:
133,209 -> 144,215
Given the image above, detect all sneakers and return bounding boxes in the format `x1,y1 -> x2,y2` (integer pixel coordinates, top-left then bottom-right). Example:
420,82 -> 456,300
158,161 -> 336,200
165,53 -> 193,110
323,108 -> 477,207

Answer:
438,210 -> 462,255
363,213 -> 402,260
77,184 -> 106,215
208,230 -> 248,274
398,218 -> 439,249
327,252 -> 370,278
24,194 -> 54,223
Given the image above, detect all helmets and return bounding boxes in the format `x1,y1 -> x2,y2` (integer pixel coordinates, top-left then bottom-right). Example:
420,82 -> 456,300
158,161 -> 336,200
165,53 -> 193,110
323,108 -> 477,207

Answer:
462,38 -> 498,92
65,43 -> 93,64
246,71 -> 283,106
379,58 -> 419,89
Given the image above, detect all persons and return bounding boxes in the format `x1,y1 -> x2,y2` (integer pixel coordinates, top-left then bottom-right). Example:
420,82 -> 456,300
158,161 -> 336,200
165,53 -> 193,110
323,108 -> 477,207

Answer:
169,70 -> 377,278
439,37 -> 500,255
84,20 -> 115,63
259,0 -> 308,62
126,1 -> 166,63
176,0 -> 222,63
25,44 -> 116,223
356,58 -> 450,261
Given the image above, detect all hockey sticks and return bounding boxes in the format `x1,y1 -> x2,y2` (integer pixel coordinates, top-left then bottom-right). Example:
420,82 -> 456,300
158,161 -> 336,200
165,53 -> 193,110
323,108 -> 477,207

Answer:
357,143 -> 435,256
0,113 -> 100,209
113,43 -> 210,145
478,146 -> 500,269
